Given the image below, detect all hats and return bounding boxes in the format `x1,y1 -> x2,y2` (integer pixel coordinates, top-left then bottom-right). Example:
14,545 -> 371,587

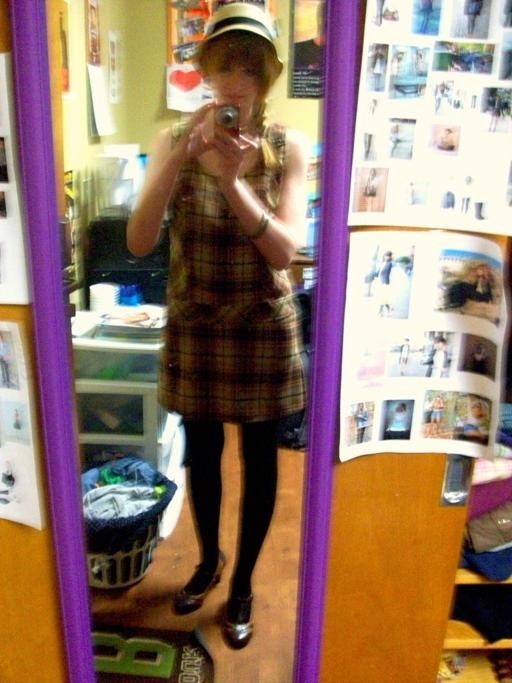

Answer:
192,3 -> 284,84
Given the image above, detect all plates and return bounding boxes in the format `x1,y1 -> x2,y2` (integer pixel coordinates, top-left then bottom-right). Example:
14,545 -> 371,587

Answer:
89,282 -> 121,311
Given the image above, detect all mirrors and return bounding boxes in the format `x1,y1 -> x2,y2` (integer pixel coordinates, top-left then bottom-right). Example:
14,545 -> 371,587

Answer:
9,0 -> 358,683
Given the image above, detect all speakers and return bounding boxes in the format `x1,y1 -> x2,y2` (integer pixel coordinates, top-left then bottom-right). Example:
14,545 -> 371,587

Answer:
86,216 -> 169,311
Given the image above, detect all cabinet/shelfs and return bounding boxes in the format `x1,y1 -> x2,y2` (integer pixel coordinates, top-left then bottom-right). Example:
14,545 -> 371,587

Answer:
434,570 -> 510,655
60,312 -> 184,512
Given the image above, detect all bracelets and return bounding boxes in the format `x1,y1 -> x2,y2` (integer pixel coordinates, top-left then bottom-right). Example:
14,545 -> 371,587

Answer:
243,209 -> 270,242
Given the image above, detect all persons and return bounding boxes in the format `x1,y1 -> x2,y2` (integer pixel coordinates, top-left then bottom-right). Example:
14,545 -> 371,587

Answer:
0,331 -> 21,505
359,0 -> 512,221
351,249 -> 495,445
125,3 -> 312,650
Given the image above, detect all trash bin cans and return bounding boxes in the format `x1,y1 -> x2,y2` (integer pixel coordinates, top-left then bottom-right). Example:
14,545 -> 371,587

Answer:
80,463 -> 177,590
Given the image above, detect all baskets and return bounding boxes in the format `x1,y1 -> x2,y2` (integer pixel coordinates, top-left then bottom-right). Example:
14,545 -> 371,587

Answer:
81,516 -> 160,587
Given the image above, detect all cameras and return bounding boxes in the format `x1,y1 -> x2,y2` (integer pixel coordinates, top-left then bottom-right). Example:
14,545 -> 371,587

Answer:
214,103 -> 240,140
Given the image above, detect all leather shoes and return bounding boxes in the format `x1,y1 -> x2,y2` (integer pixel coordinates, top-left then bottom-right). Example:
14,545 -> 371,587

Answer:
170,549 -> 226,616
225,592 -> 256,649
